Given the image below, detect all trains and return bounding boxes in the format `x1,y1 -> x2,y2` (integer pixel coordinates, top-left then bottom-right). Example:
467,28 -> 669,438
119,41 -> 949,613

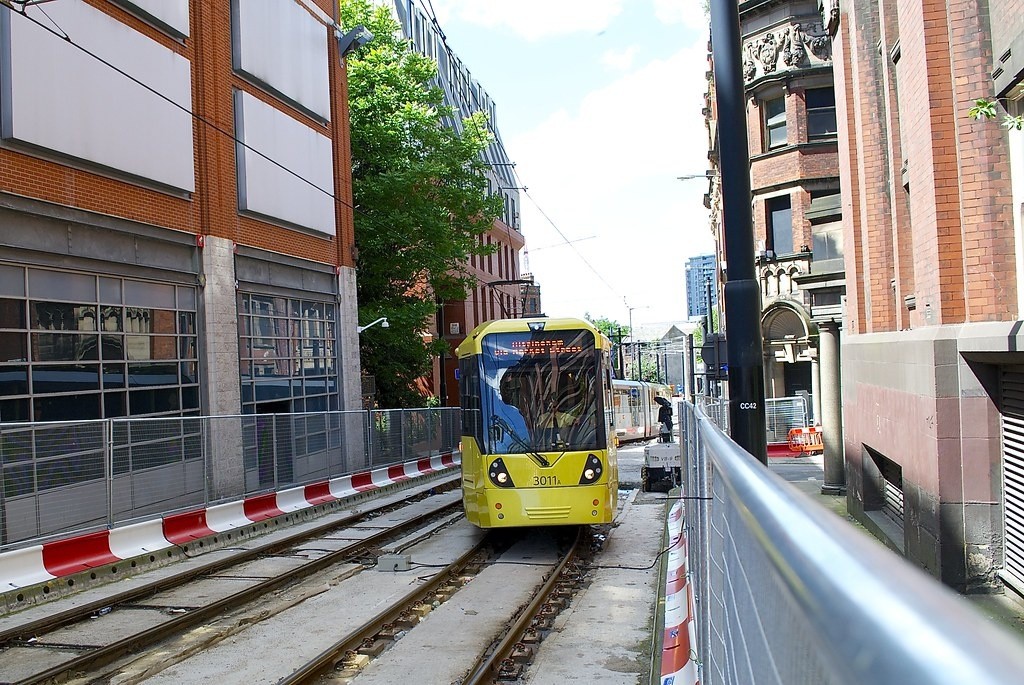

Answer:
450,317 -> 673,530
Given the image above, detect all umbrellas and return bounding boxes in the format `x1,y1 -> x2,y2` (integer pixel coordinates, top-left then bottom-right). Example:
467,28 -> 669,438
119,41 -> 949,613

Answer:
654,395 -> 672,406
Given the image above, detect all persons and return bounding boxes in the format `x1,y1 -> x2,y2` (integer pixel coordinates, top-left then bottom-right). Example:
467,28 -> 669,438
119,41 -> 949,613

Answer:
537,400 -> 576,428
657,403 -> 675,443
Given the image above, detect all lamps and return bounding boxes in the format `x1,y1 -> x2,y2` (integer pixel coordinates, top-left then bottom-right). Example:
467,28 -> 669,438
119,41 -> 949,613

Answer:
357,317 -> 389,333
